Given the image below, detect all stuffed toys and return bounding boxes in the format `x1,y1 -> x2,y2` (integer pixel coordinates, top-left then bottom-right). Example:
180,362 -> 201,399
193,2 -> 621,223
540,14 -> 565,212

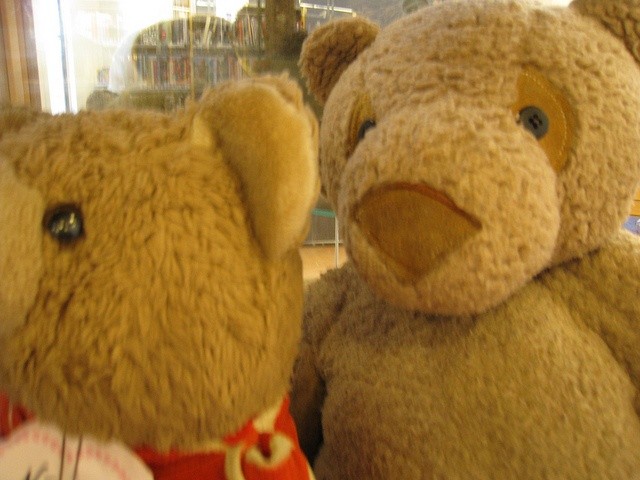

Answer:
0,74 -> 318,480
290,0 -> 639,479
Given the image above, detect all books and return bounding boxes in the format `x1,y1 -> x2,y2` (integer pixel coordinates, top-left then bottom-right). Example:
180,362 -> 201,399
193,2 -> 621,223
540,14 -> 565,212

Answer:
76,3 -> 271,102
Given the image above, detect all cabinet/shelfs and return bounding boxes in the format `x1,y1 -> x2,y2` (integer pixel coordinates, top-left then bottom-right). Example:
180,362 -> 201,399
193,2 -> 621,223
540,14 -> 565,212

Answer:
67,8 -> 249,109
